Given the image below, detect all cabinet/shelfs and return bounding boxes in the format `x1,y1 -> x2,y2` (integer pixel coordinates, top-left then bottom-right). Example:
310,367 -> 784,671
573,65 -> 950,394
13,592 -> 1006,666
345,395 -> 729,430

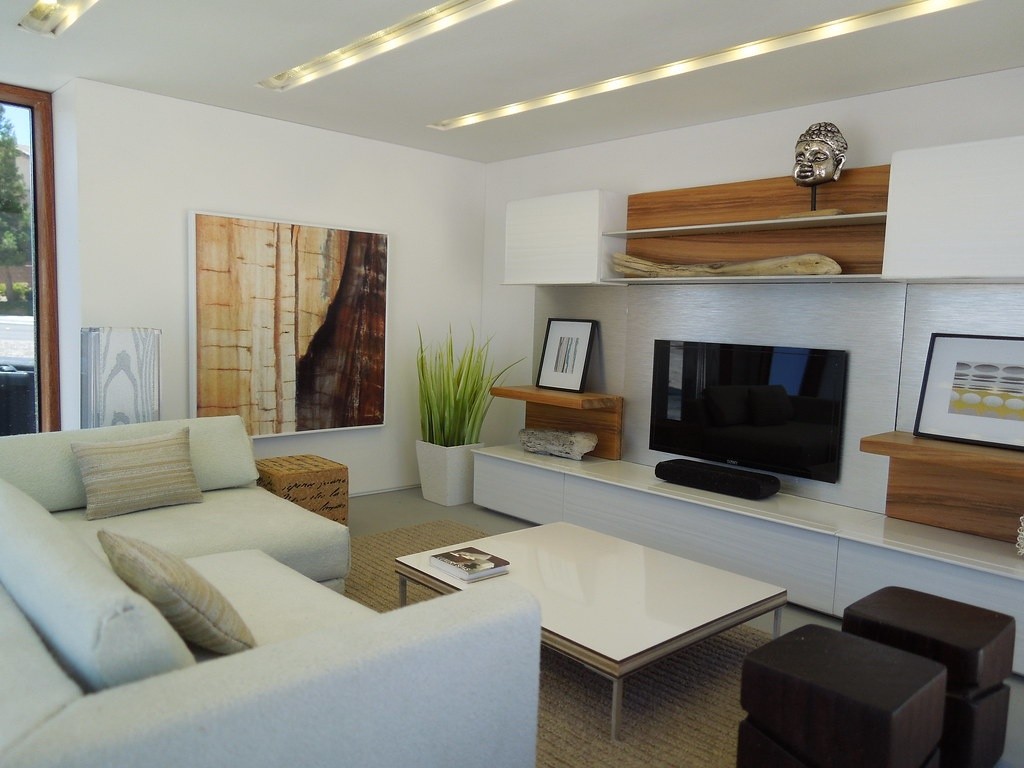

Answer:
470,445 -> 1024,678
505,136 -> 1024,288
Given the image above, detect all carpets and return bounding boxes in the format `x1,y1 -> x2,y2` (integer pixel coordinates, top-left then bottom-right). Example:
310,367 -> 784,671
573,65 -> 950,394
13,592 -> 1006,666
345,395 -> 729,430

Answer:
315,517 -> 780,768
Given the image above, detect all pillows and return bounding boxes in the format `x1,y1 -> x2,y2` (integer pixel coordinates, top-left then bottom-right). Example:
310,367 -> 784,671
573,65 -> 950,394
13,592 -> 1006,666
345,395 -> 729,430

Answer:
1,482 -> 198,690
96,527 -> 254,654
66,422 -> 204,520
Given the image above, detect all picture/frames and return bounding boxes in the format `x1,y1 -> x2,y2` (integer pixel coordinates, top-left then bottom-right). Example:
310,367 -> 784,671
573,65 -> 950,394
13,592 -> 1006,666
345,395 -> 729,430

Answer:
534,316 -> 597,392
912,332 -> 1024,449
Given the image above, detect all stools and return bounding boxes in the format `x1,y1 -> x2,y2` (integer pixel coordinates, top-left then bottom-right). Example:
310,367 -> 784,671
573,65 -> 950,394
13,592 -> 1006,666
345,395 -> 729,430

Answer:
738,586 -> 1015,768
254,452 -> 349,528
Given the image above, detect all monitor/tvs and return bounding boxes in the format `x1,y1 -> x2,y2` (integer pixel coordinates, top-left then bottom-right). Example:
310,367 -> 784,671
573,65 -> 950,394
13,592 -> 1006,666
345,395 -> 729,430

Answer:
648,340 -> 850,484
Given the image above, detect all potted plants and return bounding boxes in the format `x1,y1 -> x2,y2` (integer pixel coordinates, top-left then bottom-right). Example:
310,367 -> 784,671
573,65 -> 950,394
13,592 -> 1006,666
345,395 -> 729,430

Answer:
411,326 -> 527,505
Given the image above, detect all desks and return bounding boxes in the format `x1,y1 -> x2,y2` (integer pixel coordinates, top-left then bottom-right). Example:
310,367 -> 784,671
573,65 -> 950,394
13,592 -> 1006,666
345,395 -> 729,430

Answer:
859,430 -> 1024,542
487,382 -> 624,461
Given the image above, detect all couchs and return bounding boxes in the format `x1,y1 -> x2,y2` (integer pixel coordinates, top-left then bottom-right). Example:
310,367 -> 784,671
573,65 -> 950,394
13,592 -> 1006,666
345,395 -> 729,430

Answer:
0,415 -> 542,768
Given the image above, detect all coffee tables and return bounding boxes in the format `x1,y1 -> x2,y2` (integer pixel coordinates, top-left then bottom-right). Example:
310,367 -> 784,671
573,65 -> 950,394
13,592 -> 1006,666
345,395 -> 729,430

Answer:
396,521 -> 791,742
